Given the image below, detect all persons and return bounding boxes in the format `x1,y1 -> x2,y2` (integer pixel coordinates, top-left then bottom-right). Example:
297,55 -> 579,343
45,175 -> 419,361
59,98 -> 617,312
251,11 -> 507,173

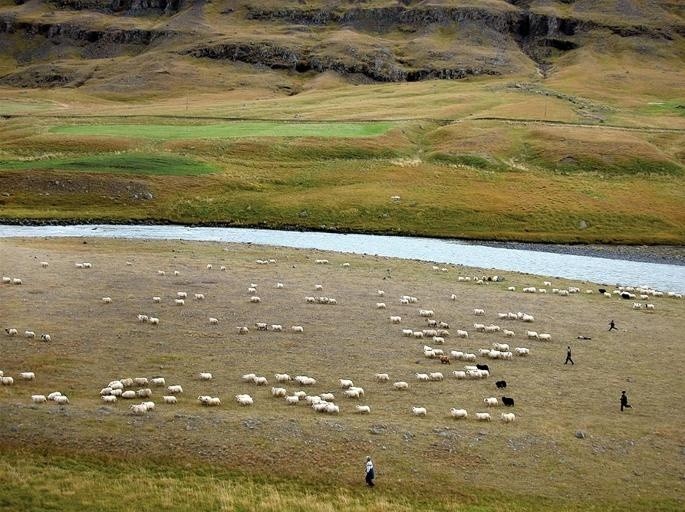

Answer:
363,454 -> 376,488
619,389 -> 633,412
564,345 -> 575,366
608,319 -> 618,332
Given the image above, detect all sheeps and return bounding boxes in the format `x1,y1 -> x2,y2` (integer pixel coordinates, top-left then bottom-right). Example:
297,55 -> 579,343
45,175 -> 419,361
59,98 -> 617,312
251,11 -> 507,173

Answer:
501,413 -> 516,423
476,412 -> 490,421
432,266 -> 505,284
495,381 -> 506,388
31,391 -> 69,405
484,397 -> 498,406
412,407 -> 426,417
199,373 -> 212,380
102,297 -> 112,303
5,328 -> 18,336
376,374 -> 389,380
394,382 -> 408,390
2,277 -> 10,283
645,304 -> 654,309
508,287 -> 516,291
136,259 -> 350,335
126,262 -> 132,266
376,303 -> 385,309
236,373 -> 364,414
75,264 -> 83,268
502,397 -> 514,406
41,334 -> 51,342
100,377 -> 183,415
13,278 -> 22,285
83,263 -> 91,268
522,281 -> 682,299
20,372 -> 35,380
198,396 -> 211,405
378,290 -> 384,296
206,398 -> 220,406
391,294 -> 553,380
41,262 -> 48,268
0,371 -> 14,385
450,408 -> 467,420
356,405 -> 370,415
632,302 -> 642,310
25,331 -> 35,338
